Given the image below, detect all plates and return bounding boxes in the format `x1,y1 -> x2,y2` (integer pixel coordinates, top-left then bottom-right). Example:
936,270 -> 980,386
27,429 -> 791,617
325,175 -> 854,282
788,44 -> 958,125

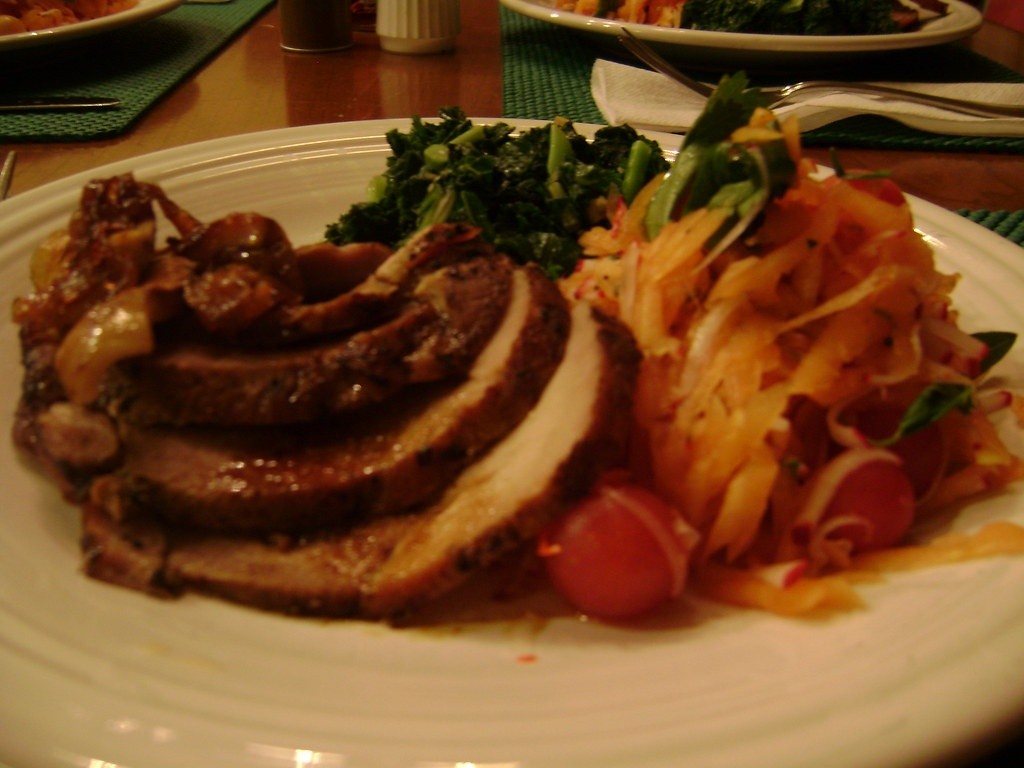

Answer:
0,1 -> 179,55
495,0 -> 982,53
1,118 -> 1024,768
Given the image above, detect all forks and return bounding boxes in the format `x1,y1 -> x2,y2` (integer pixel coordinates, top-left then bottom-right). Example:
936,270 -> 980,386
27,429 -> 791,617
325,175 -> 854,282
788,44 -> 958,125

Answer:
616,26 -> 1024,120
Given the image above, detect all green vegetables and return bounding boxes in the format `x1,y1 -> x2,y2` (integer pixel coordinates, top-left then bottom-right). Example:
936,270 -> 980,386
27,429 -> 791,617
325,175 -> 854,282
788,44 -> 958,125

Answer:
323,68 -> 1017,450
592,0 -> 916,35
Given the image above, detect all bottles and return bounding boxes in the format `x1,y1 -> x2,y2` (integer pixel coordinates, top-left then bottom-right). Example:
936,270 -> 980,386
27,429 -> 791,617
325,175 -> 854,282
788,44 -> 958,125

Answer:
376,0 -> 459,54
278,1 -> 352,52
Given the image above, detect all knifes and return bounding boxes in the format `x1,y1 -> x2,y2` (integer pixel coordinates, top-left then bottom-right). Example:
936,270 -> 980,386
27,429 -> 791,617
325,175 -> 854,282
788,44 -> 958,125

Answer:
0,95 -> 123,110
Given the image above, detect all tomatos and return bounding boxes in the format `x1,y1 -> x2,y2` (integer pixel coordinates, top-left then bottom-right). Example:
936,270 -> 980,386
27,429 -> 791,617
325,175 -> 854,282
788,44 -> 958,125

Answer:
831,460 -> 914,553
537,481 -> 695,616
